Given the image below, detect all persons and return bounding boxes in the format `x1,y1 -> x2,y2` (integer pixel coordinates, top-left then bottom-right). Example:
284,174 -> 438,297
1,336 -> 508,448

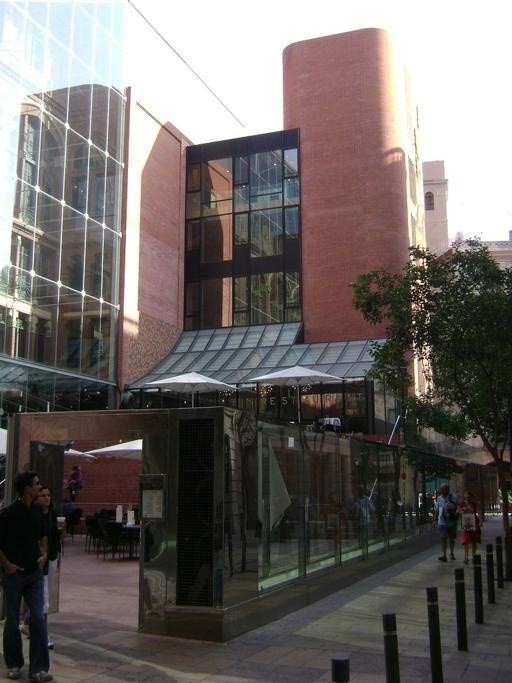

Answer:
62,496 -> 75,528
432,483 -> 482,564
320,484 -> 376,564
66,465 -> 85,502
0,471 -> 61,681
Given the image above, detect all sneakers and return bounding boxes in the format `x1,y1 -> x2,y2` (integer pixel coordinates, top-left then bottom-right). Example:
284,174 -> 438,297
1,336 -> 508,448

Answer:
439,556 -> 446,562
7,667 -> 21,678
28,671 -> 52,682
464,559 -> 468,563
19,625 -> 29,634
451,555 -> 455,560
47,637 -> 53,647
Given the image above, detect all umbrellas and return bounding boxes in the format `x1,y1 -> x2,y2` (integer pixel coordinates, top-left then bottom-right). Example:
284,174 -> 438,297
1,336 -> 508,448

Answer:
64,438 -> 142,460
248,365 -> 347,423
143,371 -> 240,408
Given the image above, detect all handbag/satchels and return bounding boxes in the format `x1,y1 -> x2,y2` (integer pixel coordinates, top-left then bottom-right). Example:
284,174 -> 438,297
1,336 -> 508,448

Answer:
48,535 -> 57,560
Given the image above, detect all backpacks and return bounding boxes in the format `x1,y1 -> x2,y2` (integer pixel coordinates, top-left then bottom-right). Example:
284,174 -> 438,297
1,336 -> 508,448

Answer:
443,494 -> 460,520
462,511 -> 476,532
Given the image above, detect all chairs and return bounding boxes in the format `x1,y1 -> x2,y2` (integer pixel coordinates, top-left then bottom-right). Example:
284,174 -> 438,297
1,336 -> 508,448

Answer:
65,506 -> 141,562
